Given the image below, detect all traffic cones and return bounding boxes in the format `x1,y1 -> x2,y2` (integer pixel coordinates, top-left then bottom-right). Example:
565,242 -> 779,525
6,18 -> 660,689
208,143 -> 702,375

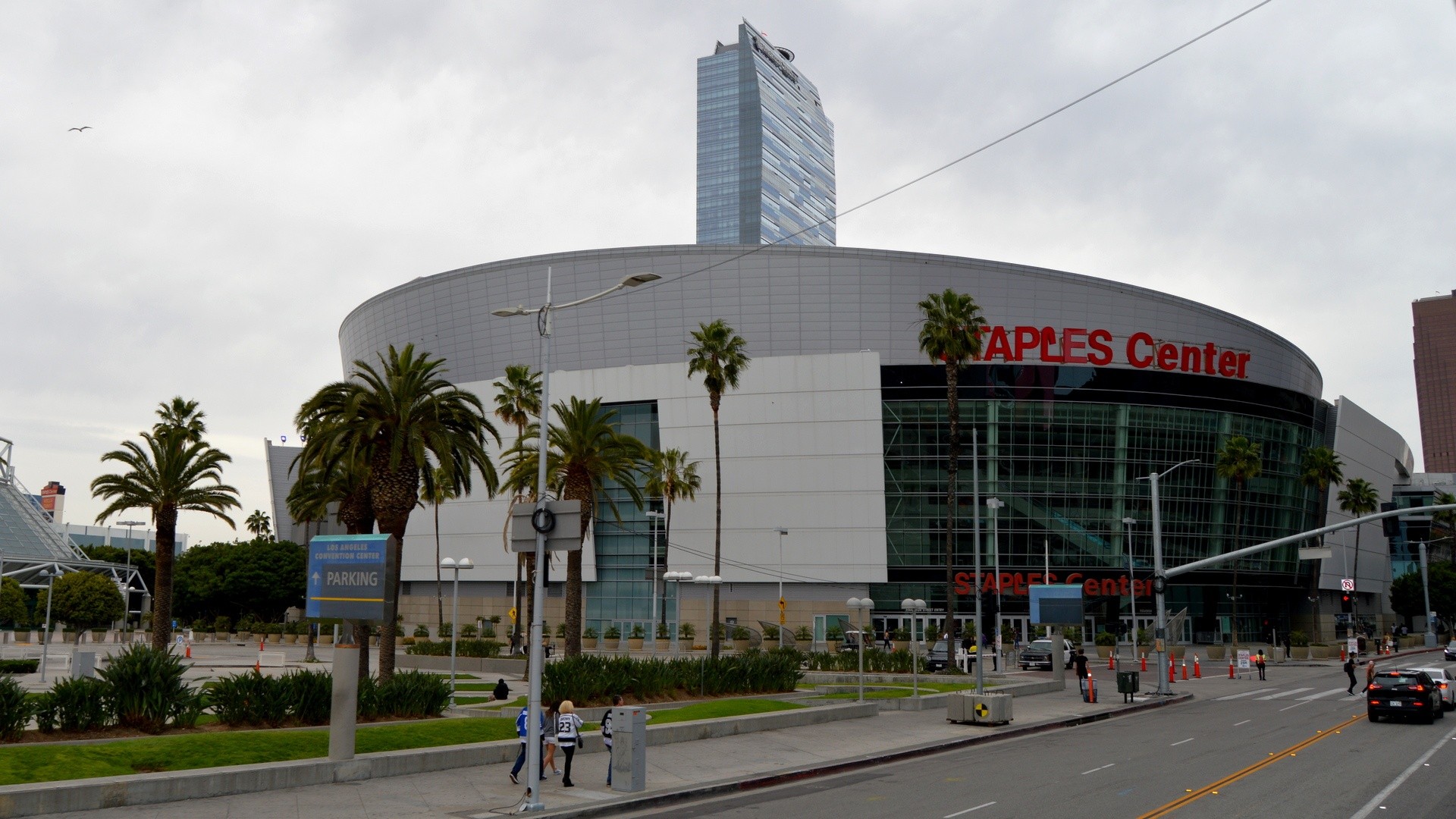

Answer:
1380,646 -> 1384,655
256,660 -> 260,673
259,637 -> 265,651
185,641 -> 192,659
1385,646 -> 1390,654
892,642 -> 896,653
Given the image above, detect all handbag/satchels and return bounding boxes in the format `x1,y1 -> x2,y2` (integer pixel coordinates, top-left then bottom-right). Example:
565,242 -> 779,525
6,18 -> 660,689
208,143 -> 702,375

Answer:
1344,663 -> 1349,672
578,736 -> 584,749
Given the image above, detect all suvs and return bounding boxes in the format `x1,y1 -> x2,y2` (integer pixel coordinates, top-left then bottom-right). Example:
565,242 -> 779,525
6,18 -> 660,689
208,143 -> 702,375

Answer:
924,638 -> 964,673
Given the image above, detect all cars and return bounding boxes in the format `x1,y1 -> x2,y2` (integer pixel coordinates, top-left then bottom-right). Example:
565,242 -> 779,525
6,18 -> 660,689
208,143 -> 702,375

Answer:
1017,637 -> 1076,671
1444,640 -> 1456,661
1399,668 -> 1456,711
1367,671 -> 1442,725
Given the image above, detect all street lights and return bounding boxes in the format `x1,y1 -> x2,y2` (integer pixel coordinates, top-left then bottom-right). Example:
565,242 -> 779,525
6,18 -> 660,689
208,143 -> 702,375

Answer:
116,521 -> 146,644
493,265 -> 662,814
1404,536 -> 1453,647
987,497 -> 1004,673
645,510 -> 666,662
901,599 -> 927,698
1135,459 -> 1201,694
846,598 -> 874,704
694,576 -> 723,661
38,570 -> 64,684
664,572 -> 693,660
1122,517 -> 1139,661
440,557 -> 474,707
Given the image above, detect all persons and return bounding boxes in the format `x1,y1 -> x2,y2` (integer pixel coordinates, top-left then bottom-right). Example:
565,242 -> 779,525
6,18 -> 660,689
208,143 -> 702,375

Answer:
1255,649 -> 1267,680
556,700 -> 584,787
493,678 -> 509,700
1072,648 -> 1091,695
1346,652 -> 1357,696
1012,630 -> 1020,649
509,633 -> 515,654
883,629 -> 891,650
1365,619 -> 1407,652
541,701 -> 563,778
375,625 -> 382,647
1360,660 -> 1375,697
508,703 -> 545,785
991,642 -> 997,671
601,694 -> 625,787
982,633 -> 988,649
1283,636 -> 1291,658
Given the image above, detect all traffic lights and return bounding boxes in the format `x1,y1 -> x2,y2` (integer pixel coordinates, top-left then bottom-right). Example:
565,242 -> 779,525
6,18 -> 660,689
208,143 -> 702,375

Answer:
1340,594 -> 1353,613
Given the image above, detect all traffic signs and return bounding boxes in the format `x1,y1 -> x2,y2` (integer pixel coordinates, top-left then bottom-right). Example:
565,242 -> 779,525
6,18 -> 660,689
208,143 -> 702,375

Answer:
306,533 -> 396,626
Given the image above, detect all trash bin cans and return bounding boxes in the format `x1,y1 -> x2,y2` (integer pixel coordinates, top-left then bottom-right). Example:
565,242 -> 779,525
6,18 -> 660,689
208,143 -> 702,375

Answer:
1273,647 -> 1285,662
1081,678 -> 1098,702
1118,671 -> 1139,693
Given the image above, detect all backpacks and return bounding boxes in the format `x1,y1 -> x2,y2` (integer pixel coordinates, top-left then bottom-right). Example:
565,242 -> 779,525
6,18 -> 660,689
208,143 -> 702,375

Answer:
1258,656 -> 1264,663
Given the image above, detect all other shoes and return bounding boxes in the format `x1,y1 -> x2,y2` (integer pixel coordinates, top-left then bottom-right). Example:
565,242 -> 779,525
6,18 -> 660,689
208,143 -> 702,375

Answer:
1347,690 -> 1354,695
563,779 -> 571,783
564,784 -> 574,787
606,782 -> 611,786
510,771 -> 519,784
540,776 -> 546,780
1360,692 -> 1364,698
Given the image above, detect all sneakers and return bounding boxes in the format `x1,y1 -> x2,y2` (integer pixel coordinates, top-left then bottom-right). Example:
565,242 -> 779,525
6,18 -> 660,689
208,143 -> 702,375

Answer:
554,769 -> 561,774
